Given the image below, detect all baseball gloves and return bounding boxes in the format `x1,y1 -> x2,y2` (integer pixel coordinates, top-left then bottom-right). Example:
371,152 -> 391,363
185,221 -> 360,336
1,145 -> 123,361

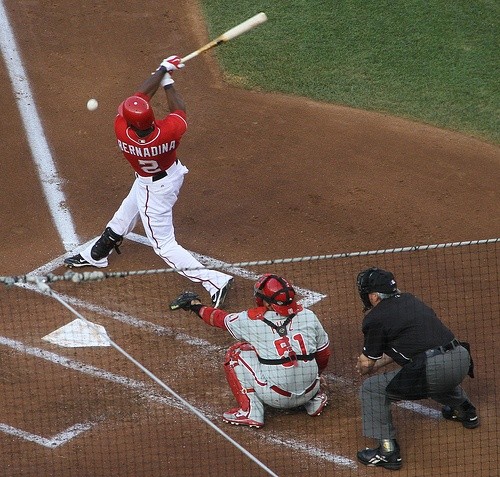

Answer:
169,290 -> 200,312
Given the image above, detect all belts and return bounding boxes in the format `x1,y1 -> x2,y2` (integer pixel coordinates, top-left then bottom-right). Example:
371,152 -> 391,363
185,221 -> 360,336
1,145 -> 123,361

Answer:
134,159 -> 181,184
414,339 -> 459,358
271,379 -> 318,397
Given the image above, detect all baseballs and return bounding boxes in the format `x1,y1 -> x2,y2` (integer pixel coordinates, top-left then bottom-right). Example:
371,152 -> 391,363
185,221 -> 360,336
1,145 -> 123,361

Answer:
86,98 -> 98,112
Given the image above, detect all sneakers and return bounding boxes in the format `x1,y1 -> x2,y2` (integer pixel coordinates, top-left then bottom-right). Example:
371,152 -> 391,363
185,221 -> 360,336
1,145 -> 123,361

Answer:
63,250 -> 108,267
222,408 -> 263,428
357,447 -> 401,469
307,393 -> 327,415
211,278 -> 233,309
441,403 -> 481,428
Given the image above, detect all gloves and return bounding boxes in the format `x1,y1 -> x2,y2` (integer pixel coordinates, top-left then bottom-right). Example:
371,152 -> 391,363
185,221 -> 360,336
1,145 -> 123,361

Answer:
151,67 -> 175,87
159,56 -> 186,71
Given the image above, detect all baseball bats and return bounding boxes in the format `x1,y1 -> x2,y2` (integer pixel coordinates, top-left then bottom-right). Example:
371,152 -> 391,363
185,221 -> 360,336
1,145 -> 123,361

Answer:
178,11 -> 268,63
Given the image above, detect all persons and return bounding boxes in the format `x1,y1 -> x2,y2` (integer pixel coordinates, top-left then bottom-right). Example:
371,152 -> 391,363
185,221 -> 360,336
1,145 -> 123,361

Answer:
63,56 -> 234,313
169,274 -> 330,428
354,268 -> 479,470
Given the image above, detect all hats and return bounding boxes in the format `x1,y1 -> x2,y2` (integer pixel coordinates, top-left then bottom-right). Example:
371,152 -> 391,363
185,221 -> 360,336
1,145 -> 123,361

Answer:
362,270 -> 397,293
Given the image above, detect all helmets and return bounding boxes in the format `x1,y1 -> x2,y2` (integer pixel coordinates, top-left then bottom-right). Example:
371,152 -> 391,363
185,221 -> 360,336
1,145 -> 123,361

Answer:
118,96 -> 155,130
254,274 -> 303,315
